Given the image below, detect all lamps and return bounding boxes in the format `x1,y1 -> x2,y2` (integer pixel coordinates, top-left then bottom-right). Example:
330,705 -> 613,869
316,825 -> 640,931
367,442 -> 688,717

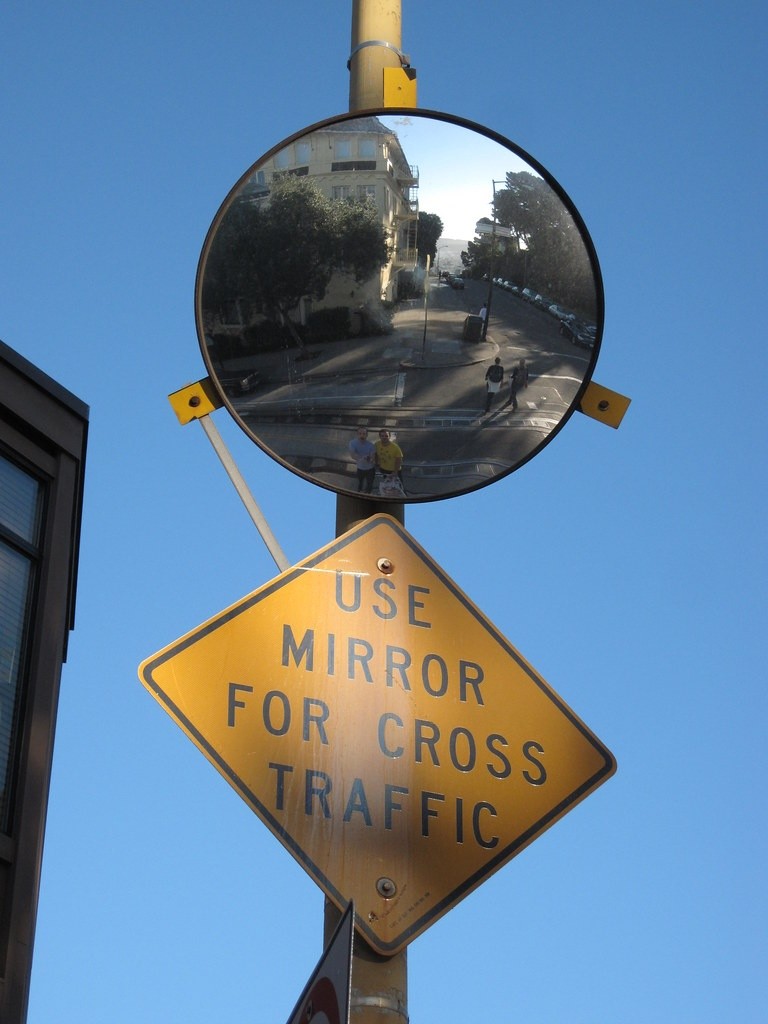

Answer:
194,106 -> 603,503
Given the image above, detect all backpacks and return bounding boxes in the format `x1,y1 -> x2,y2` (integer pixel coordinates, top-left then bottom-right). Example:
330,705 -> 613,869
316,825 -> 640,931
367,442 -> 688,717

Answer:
516,366 -> 527,383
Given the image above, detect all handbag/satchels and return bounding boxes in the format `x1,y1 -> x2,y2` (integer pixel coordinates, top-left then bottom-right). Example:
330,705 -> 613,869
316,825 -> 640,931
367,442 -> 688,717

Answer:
379,474 -> 406,497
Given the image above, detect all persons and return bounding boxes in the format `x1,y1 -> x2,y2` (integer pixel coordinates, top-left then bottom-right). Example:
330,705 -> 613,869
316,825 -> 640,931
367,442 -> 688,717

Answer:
375,429 -> 407,495
485,356 -> 504,401
506,359 -> 528,411
479,303 -> 487,322
348,426 -> 376,494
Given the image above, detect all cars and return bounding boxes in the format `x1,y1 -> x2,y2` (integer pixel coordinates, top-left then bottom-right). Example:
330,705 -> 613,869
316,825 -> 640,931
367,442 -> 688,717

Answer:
442,271 -> 465,289
214,369 -> 261,396
481,273 -> 596,351
